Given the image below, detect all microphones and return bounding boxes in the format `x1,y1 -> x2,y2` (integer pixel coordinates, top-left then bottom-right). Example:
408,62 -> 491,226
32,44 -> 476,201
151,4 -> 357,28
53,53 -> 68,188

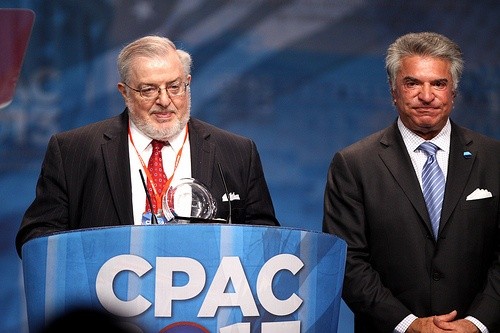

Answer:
139,169 -> 155,225
217,163 -> 232,224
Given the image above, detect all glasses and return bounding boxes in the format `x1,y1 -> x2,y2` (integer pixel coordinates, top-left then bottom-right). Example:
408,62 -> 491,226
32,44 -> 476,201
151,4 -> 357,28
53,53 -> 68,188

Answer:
123,81 -> 189,100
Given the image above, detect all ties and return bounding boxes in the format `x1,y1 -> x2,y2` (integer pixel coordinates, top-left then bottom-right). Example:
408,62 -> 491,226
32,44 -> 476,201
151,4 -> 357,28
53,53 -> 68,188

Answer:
146,139 -> 174,217
417,142 -> 446,245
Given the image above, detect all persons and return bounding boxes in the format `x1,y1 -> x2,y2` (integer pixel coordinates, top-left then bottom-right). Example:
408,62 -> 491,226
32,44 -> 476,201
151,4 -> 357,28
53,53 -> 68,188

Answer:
323,32 -> 500,333
15,35 -> 279,260
